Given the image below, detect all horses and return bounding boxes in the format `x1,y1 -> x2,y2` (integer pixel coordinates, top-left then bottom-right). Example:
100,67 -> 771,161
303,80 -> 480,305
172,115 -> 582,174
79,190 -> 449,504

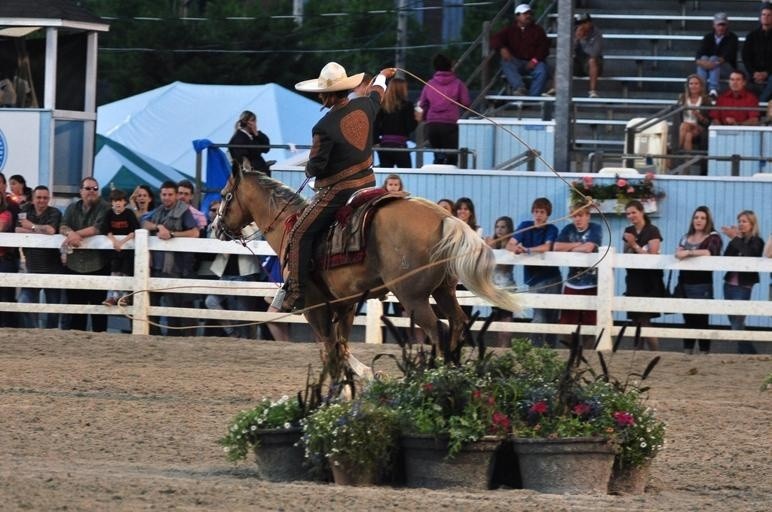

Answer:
210,154 -> 526,400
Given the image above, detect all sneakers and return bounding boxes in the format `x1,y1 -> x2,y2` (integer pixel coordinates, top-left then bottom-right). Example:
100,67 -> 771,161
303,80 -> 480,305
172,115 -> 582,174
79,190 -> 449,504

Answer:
588,90 -> 598,97
102,297 -> 115,305
542,89 -> 555,96
118,297 -> 129,305
513,86 -> 527,96
709,89 -> 718,98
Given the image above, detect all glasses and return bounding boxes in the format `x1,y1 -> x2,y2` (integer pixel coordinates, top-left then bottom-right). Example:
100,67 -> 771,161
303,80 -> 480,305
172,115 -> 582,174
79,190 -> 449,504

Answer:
84,186 -> 98,190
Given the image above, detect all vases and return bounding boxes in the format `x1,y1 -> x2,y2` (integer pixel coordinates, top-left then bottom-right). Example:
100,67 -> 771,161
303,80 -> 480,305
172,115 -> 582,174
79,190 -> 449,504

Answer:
568,198 -> 657,214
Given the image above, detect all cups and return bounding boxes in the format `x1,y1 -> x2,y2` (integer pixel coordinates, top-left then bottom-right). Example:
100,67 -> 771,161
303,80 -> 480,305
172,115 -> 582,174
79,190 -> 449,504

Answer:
415,107 -> 423,123
18,213 -> 28,221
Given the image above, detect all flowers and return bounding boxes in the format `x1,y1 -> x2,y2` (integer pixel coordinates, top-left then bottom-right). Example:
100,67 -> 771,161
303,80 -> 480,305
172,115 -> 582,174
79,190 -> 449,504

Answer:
568,172 -> 665,210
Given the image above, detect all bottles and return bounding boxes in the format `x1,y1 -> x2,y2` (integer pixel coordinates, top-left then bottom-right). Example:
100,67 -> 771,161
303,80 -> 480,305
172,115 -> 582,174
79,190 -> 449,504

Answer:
61,242 -> 68,263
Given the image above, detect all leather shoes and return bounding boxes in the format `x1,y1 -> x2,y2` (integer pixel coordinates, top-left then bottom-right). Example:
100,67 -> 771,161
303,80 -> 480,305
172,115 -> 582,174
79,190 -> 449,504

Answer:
264,296 -> 304,314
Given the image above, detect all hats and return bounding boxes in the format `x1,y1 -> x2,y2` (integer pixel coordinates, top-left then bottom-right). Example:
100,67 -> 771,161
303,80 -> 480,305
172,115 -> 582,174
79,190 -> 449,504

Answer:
574,13 -> 590,25
715,13 -> 727,25
295,62 -> 365,93
515,4 -> 531,14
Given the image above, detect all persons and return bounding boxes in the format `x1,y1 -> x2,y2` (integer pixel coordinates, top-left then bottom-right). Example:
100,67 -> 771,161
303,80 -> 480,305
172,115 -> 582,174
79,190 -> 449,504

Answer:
374,77 -> 418,168
678,75 -> 712,164
491,3 -> 551,101
0,173 -> 298,345
708,71 -> 759,126
695,12 -> 739,105
765,232 -> 772,311
553,200 -> 605,345
437,197 -> 514,320
279,61 -> 397,314
506,197 -> 563,349
229,110 -> 271,177
675,206 -> 722,354
414,56 -> 471,165
623,201 -> 668,351
548,12 -> 604,98
742,3 -> 772,102
719,210 -> 765,355
384,175 -> 404,194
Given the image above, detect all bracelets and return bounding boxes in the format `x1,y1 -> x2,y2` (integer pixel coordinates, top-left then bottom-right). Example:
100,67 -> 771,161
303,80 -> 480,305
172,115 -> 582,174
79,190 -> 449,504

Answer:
714,60 -> 721,66
526,248 -> 530,254
687,250 -> 693,255
700,117 -> 704,122
531,59 -> 539,65
